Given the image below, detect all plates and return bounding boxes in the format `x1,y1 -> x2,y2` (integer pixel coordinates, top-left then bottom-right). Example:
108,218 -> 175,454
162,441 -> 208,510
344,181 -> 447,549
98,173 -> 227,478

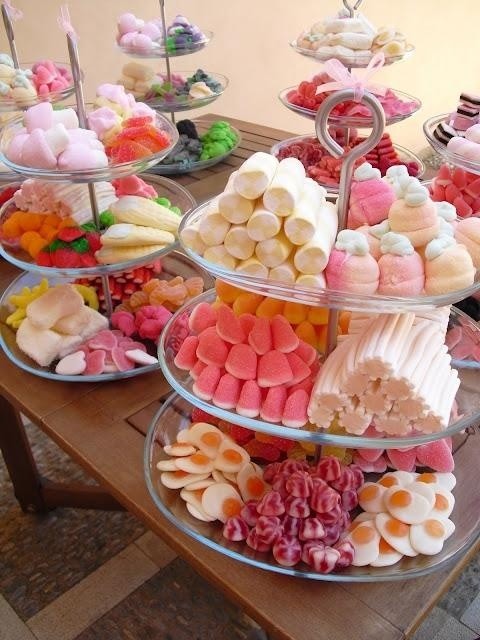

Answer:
0,102 -> 179,183
116,31 -> 213,58
270,135 -> 426,197
452,354 -> 478,370
107,71 -> 229,112
422,112 -> 480,178
177,194 -> 480,313
144,377 -> 480,582
289,35 -> 415,66
0,60 -> 85,110
0,249 -> 217,382
0,173 -> 199,277
278,85 -> 422,126
157,286 -> 480,448
136,119 -> 242,174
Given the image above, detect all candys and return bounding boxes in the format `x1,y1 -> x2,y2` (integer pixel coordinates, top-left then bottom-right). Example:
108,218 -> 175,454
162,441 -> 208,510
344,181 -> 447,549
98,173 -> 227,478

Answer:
0,175 -> 182,269
432,91 -> 480,165
160,118 -> 238,173
115,14 -> 207,57
156,407 -> 456,572
175,278 -> 480,436
5,83 -> 170,169
287,73 -> 416,118
296,8 -> 407,66
5,261 -> 204,376
0,53 -> 73,108
116,62 -> 224,110
275,128 -> 419,185
181,151 -> 480,296
